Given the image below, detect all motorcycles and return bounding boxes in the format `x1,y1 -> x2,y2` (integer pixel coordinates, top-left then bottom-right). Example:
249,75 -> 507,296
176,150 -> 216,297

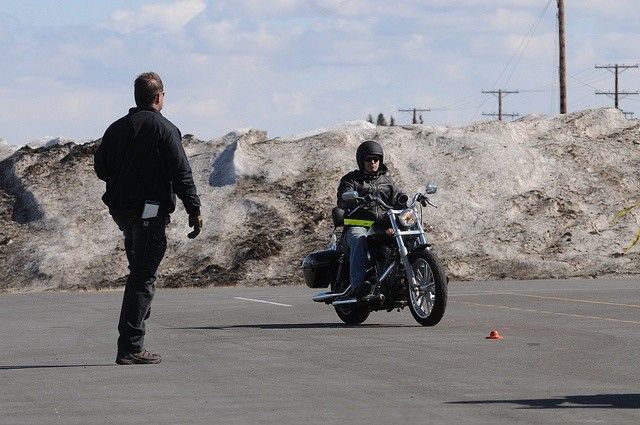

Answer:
301,184 -> 447,326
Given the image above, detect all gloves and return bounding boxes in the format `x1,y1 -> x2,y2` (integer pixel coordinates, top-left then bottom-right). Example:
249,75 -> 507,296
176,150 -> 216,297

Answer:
187,211 -> 202,239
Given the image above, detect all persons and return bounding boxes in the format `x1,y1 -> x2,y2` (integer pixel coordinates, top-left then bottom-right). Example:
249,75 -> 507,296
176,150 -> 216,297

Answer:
94,72 -> 202,365
337,140 -> 433,298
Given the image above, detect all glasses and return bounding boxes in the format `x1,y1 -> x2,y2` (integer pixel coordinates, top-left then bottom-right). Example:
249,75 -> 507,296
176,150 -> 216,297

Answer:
161,92 -> 165,96
364,156 -> 379,162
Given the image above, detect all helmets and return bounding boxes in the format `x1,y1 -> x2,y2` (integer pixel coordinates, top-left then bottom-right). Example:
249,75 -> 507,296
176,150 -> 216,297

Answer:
356,141 -> 383,171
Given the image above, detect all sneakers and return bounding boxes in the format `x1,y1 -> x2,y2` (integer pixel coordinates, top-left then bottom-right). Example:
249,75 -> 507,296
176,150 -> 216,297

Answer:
115,348 -> 162,364
354,281 -> 371,300
